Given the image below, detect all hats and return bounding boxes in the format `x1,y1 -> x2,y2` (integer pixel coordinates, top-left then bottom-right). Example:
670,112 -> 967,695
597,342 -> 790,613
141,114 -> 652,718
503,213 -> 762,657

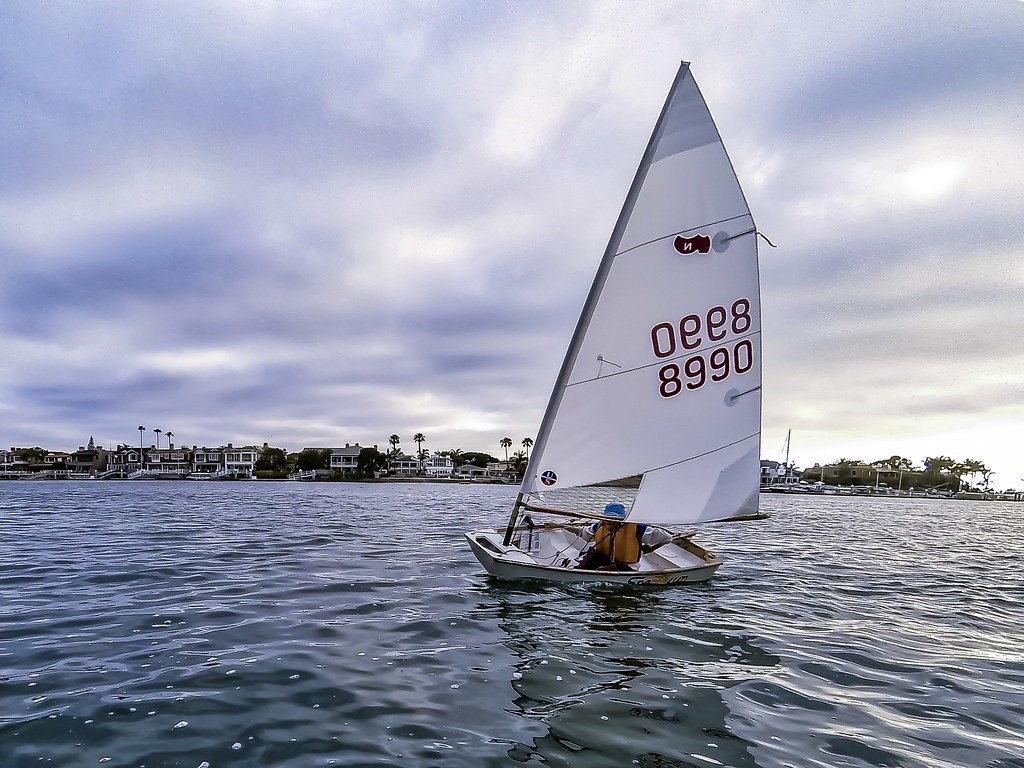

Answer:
602,502 -> 626,519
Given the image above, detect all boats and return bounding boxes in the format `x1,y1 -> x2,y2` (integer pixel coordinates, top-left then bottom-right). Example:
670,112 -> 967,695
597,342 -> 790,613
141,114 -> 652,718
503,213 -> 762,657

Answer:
771,480 -> 838,494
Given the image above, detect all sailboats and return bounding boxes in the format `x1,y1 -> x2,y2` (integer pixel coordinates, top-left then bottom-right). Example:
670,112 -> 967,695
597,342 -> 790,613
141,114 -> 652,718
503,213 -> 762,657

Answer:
463,60 -> 773,582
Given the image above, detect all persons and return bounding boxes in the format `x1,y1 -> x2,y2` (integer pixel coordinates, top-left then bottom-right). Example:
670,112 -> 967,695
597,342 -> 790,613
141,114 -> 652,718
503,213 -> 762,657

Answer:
544,502 -> 672,572
